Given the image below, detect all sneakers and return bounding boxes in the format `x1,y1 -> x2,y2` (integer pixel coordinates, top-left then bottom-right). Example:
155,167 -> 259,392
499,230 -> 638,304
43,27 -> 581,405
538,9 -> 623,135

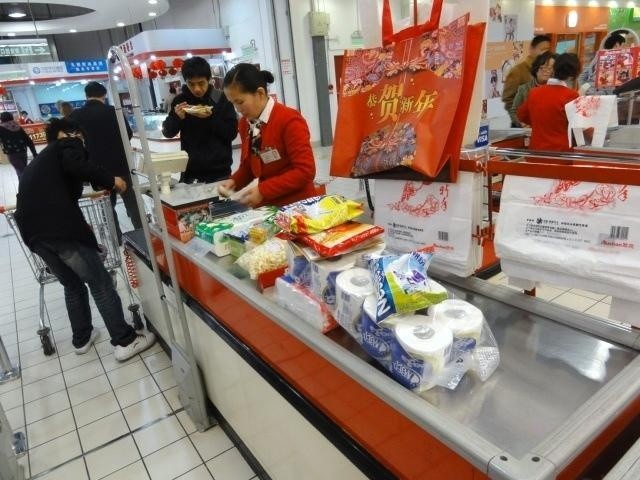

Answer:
111,330 -> 156,362
70,325 -> 101,355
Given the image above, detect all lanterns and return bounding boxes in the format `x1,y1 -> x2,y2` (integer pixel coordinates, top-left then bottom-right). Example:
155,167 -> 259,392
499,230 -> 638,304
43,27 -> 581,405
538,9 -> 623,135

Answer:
119,58 -> 184,80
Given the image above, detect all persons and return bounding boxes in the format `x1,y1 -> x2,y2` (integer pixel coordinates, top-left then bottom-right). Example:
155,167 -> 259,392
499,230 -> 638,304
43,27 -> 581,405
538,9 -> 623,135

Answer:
14,117 -> 156,361
67,81 -> 142,245
17,110 -> 34,124
502,33 -> 627,153
60,103 -> 72,118
217,64 -> 316,209
0,112 -> 38,180
162,57 -> 240,183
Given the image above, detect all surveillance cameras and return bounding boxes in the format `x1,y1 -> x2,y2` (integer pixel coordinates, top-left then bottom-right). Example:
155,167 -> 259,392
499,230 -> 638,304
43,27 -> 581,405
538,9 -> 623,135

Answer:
110,53 -> 119,65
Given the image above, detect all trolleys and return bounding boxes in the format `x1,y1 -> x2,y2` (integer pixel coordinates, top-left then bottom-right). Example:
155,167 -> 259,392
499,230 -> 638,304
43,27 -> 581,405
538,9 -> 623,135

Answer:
0,184 -> 147,360
